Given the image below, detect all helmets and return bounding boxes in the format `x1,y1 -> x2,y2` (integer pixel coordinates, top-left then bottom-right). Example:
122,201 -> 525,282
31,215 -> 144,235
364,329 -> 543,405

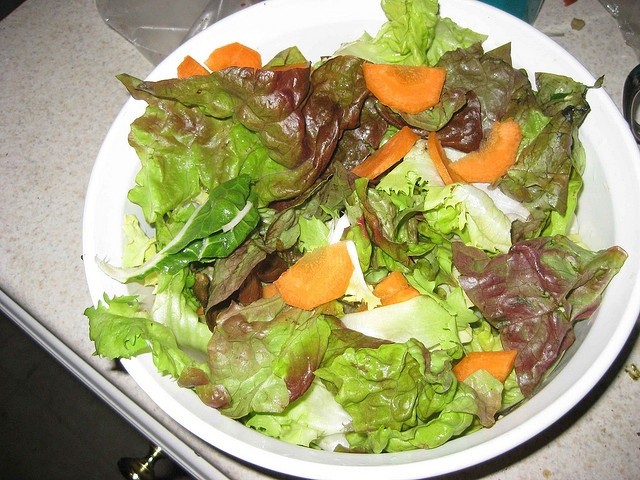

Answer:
82,0 -> 640,480
